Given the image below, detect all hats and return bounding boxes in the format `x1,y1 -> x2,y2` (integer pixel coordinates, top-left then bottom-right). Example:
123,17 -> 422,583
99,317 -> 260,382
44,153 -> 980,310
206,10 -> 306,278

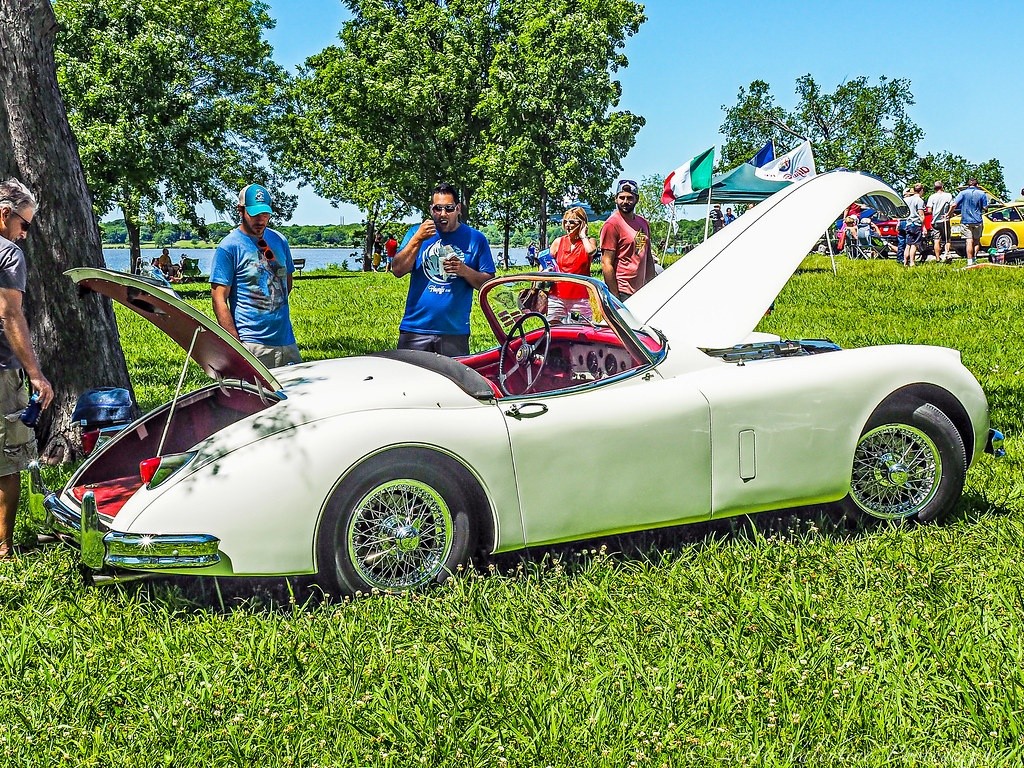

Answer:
903,188 -> 910,195
498,252 -> 503,256
714,203 -> 721,209
616,180 -> 639,195
908,188 -> 915,194
237,183 -> 274,217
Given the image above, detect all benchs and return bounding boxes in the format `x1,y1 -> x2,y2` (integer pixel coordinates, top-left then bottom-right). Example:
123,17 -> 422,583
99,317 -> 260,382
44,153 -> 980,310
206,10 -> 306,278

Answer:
292,259 -> 305,277
498,259 -> 517,265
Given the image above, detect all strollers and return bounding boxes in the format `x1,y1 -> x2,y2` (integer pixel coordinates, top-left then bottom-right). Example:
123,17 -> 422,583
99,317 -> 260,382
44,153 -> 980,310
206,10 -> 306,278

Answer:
135,257 -> 164,279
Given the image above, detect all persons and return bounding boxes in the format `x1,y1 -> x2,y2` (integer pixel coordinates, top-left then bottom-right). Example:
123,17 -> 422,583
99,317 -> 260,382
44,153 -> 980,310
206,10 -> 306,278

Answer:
153,249 -> 190,283
209,182 -> 304,369
392,184 -> 496,357
497,242 -> 540,269
836,178 -> 1024,268
372,233 -> 399,274
599,179 -> 655,302
0,178 -> 55,562
531,207 -> 596,324
654,204 -> 753,276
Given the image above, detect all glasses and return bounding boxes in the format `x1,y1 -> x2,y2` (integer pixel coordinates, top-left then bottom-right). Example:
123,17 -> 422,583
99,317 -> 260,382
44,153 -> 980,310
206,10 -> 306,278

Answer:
563,219 -> 580,225
619,180 -> 637,187
432,203 -> 457,213
11,210 -> 31,231
257,237 -> 276,262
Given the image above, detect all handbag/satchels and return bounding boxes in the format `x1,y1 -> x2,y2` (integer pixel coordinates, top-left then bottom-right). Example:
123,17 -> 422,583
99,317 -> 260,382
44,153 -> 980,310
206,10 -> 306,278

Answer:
72,387 -> 133,425
518,287 -> 549,315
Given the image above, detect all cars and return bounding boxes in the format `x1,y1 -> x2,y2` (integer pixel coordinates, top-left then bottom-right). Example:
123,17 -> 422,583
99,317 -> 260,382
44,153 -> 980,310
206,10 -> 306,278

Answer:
950,185 -> 1024,257
873,205 -> 933,243
37,168 -> 1006,595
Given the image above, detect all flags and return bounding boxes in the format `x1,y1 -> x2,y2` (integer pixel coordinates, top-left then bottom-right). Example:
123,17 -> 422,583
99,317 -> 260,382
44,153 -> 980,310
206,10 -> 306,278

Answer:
749,143 -> 775,169
659,146 -> 715,204
754,142 -> 816,184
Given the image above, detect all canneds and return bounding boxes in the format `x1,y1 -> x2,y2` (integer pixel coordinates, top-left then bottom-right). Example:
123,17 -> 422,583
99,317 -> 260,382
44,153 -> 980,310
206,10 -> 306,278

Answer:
21,394 -> 45,428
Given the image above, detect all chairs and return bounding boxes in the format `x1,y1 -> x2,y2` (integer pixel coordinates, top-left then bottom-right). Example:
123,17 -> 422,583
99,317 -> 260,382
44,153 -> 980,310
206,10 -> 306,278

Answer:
842,222 -> 891,260
135,256 -> 201,284
992,212 -> 1003,220
1010,211 -> 1023,221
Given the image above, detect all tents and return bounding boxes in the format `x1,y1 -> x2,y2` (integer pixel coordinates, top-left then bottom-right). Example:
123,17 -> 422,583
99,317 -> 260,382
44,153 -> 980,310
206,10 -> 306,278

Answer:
660,162 -> 836,276
546,201 -> 610,223
